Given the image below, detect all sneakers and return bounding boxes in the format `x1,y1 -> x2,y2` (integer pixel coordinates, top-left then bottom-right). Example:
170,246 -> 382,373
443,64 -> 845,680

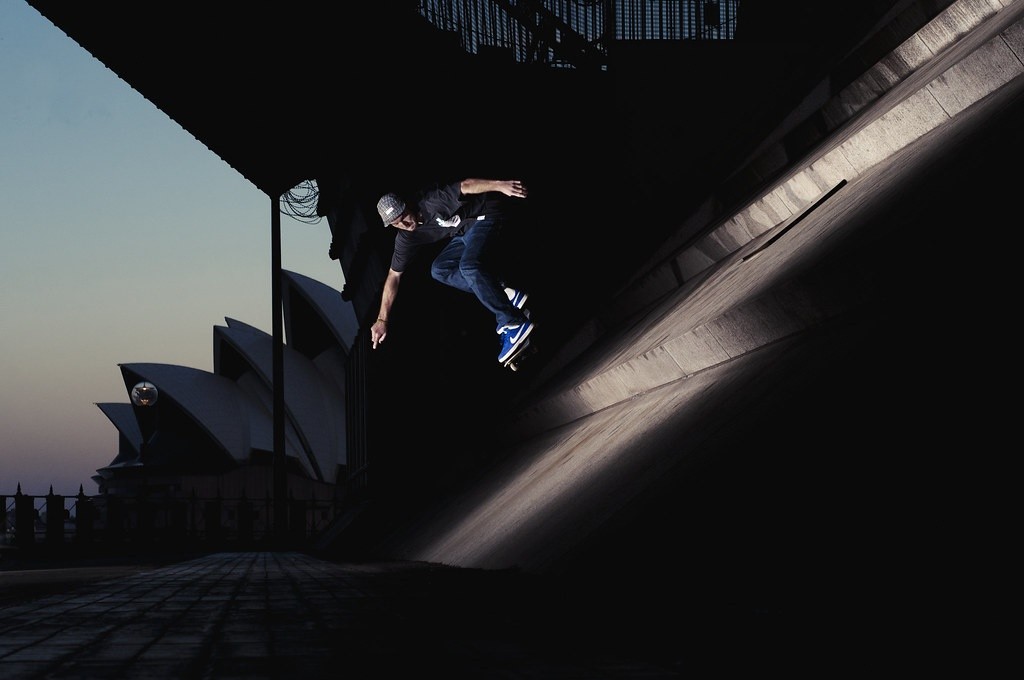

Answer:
496,288 -> 528,334
497,314 -> 534,363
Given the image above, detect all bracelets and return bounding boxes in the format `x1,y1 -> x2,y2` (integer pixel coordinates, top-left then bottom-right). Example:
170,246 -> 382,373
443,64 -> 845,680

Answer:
376,319 -> 388,323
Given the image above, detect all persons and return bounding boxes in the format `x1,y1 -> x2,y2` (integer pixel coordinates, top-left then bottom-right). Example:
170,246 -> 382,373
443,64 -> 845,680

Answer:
371,178 -> 534,363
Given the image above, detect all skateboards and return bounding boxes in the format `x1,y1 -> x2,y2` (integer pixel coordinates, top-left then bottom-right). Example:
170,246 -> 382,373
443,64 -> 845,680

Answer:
503,309 -> 531,372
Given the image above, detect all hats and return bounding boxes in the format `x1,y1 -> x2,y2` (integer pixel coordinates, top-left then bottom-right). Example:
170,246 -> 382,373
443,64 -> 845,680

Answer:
376,194 -> 406,227
